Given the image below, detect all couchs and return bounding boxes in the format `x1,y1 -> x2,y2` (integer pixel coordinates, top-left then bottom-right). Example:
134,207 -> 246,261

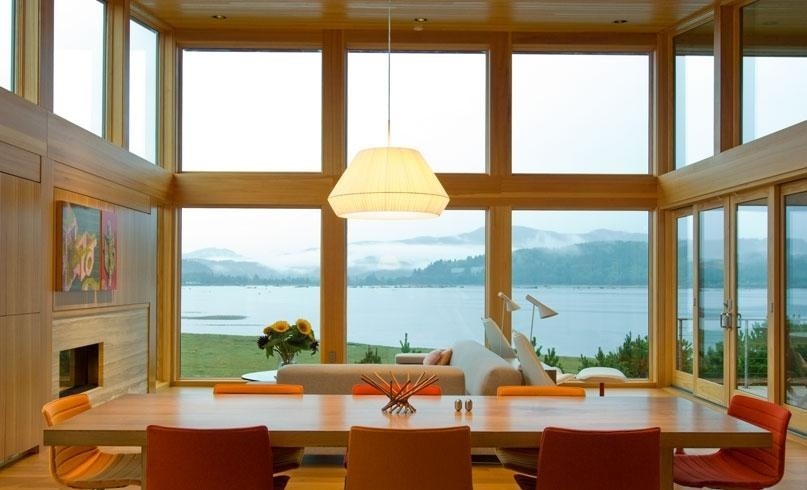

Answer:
276,318 -> 629,395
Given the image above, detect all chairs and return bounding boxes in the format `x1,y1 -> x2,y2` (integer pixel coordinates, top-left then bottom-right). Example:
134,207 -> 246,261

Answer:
673,394 -> 792,487
213,383 -> 305,474
513,426 -> 662,490
491,386 -> 586,476
40,392 -> 144,489
343,424 -> 474,490
146,424 -> 291,490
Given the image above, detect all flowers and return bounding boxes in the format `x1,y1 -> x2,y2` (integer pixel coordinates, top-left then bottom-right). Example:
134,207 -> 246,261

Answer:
256,318 -> 319,351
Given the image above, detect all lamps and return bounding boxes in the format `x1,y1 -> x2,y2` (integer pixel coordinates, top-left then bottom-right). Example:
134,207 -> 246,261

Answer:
496,290 -> 519,328
329,1 -> 450,226
524,294 -> 559,341
